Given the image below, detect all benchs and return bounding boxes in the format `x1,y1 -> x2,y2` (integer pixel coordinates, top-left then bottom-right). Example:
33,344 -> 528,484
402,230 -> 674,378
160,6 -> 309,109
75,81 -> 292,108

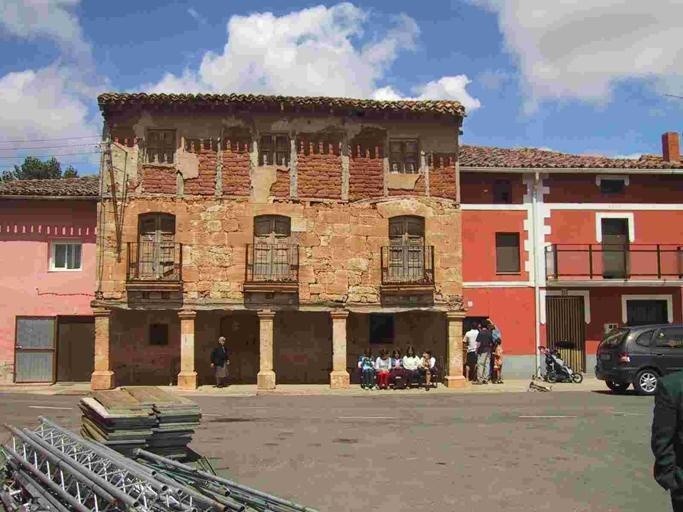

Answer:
357,361 -> 438,389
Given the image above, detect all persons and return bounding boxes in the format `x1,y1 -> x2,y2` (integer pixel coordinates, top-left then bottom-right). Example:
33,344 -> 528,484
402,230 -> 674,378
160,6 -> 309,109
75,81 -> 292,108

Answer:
649,369 -> 682,512
357,345 -> 436,391
208,336 -> 230,388
462,318 -> 505,384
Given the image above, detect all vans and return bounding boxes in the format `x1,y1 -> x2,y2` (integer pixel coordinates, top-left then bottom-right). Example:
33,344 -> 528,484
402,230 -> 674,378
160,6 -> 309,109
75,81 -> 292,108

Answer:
595,321 -> 682,395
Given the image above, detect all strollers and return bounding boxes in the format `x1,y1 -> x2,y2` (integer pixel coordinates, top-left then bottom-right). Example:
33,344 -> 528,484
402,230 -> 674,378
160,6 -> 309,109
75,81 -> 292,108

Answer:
539,346 -> 582,383
